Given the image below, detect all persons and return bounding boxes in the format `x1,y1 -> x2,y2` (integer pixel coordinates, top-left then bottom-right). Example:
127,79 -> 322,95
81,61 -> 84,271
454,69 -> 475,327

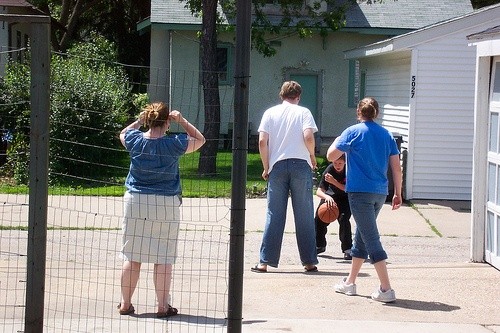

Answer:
327,97 -> 402,302
314,153 -> 353,260
120,103 -> 206,318
250,81 -> 319,273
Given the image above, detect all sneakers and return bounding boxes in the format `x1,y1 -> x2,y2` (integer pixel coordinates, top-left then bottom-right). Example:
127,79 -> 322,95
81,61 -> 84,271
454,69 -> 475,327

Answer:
335,278 -> 356,295
370,286 -> 396,302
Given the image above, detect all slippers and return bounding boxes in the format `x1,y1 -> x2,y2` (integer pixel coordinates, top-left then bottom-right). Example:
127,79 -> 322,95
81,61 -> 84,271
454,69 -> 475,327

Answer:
251,264 -> 267,273
307,267 -> 318,272
119,303 -> 134,315
157,304 -> 178,318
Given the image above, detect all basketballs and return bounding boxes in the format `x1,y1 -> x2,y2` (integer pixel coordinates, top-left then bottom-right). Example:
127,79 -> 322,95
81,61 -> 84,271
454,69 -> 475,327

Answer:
318,202 -> 339,224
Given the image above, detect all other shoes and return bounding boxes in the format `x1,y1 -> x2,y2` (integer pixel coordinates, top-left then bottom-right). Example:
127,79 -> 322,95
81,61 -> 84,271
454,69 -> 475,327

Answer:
344,253 -> 352,260
316,246 -> 325,254
